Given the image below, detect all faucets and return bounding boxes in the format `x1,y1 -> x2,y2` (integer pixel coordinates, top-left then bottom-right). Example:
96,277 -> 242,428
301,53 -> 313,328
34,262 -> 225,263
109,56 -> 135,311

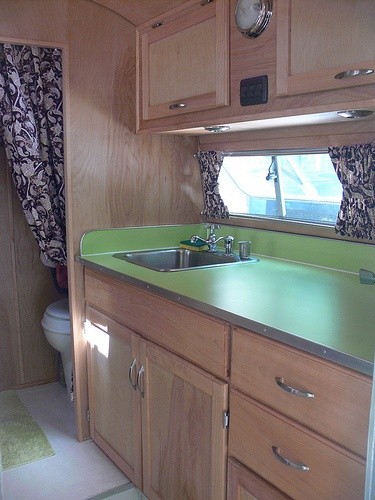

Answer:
191,236 -> 209,245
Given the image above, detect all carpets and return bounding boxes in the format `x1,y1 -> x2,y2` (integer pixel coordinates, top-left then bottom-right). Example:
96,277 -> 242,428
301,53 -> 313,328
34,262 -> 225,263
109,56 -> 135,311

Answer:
0,389 -> 54,473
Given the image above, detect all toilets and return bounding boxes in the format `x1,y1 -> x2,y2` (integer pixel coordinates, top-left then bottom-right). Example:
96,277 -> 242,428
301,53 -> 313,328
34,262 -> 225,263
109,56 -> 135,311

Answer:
41,298 -> 74,397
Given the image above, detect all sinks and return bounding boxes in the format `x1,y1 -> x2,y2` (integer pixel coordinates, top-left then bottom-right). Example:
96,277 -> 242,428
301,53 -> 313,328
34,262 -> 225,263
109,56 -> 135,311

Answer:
125,249 -> 239,269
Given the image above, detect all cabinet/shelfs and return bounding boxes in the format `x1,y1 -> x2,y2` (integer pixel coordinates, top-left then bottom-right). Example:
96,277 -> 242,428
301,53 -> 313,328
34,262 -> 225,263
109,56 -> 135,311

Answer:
74,255 -> 375,500
134,0 -> 375,137
